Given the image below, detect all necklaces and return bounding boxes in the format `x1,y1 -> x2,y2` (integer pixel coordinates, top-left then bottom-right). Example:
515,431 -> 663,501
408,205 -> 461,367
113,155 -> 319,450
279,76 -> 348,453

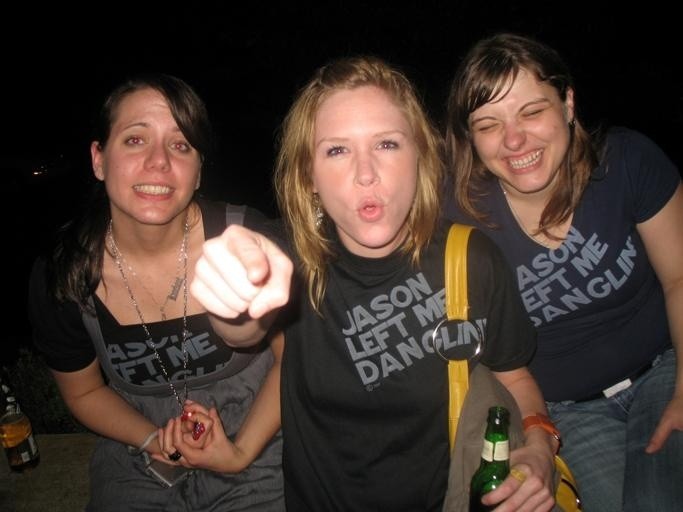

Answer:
105,231 -> 205,441
498,181 -> 562,248
103,210 -> 189,321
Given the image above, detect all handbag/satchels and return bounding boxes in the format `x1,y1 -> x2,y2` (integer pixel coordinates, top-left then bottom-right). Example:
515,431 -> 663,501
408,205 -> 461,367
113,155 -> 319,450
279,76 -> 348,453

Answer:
441,222 -> 580,512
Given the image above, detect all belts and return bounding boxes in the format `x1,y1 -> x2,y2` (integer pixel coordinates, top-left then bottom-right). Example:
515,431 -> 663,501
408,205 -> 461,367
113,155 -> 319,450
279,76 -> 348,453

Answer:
575,356 -> 652,403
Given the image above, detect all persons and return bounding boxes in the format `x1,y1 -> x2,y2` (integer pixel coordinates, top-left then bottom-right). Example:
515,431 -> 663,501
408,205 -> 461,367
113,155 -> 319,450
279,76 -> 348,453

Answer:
438,30 -> 682,511
190,56 -> 562,511
41,74 -> 291,512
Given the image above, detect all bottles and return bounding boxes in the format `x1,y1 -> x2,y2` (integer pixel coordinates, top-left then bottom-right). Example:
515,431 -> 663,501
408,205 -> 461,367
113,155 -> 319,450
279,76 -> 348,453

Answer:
0,382 -> 41,472
467,406 -> 512,512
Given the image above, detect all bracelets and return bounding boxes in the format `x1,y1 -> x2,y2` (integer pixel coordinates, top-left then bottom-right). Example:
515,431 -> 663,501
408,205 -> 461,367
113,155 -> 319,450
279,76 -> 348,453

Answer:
518,411 -> 561,455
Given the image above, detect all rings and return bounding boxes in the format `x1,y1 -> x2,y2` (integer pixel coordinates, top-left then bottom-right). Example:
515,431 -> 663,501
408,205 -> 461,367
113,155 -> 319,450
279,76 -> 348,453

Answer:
166,449 -> 181,462
511,468 -> 528,485
